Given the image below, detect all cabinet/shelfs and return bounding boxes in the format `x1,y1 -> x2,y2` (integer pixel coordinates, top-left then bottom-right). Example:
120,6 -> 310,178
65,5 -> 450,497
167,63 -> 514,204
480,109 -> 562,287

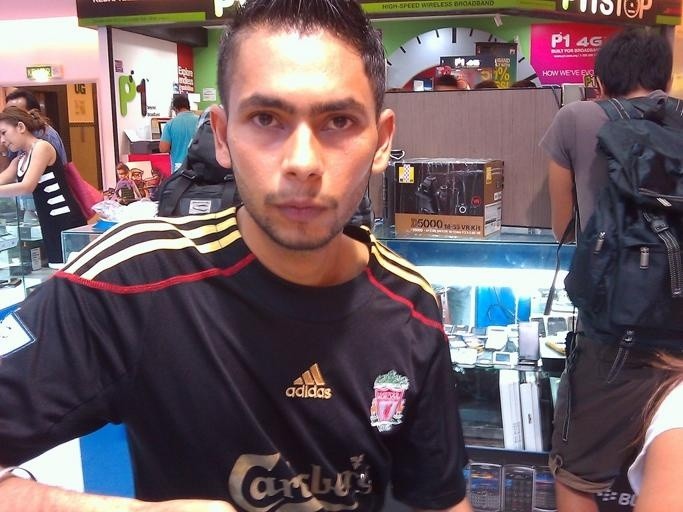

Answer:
0,193 -> 56,326
59,219 -> 578,512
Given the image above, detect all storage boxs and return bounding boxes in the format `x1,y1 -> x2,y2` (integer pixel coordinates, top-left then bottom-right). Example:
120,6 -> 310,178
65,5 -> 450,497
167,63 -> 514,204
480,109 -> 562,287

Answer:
394,157 -> 504,238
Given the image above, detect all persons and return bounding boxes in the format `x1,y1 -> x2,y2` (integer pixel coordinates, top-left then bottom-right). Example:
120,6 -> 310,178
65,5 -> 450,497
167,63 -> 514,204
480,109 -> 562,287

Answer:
597,347 -> 682,511
114,163 -> 142,205
0,1 -> 474,512
0,104 -> 92,263
1,89 -> 68,220
433,74 -> 459,91
537,24 -> 682,512
157,94 -> 204,175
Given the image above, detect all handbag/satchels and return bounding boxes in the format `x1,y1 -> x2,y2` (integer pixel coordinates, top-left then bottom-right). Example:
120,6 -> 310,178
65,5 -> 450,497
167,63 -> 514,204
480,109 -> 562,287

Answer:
63,161 -> 104,220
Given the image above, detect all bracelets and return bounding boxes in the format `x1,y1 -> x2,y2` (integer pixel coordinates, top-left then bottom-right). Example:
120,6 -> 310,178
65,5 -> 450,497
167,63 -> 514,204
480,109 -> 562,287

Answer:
0,465 -> 36,481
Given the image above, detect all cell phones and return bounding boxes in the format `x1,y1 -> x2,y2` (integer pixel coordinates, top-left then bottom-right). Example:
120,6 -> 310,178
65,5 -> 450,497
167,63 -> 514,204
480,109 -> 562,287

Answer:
0,278 -> 22,287
502,464 -> 534,512
534,466 -> 558,512
442,316 -> 577,368
470,463 -> 502,512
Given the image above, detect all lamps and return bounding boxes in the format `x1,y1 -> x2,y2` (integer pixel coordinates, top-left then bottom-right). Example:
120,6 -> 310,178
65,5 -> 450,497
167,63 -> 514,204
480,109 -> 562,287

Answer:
493,14 -> 504,28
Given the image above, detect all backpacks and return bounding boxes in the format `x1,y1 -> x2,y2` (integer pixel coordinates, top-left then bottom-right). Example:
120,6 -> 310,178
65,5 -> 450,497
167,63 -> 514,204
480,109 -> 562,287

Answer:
562,97 -> 683,385
155,120 -> 240,217
